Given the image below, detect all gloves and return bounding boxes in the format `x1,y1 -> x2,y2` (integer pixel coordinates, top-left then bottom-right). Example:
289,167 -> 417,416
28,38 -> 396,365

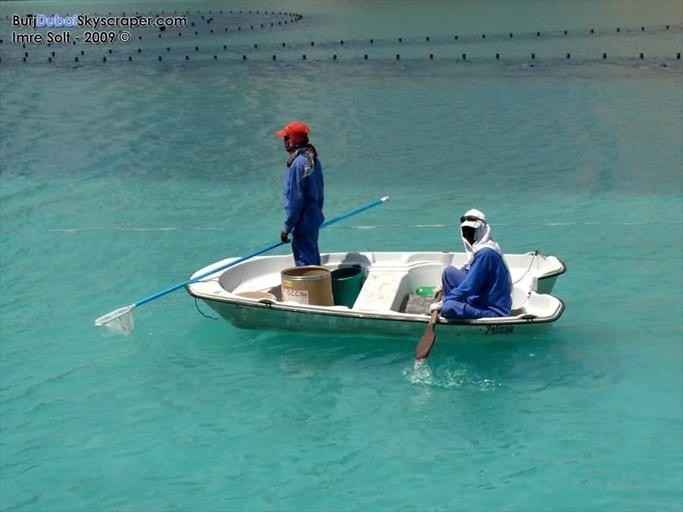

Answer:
429,301 -> 443,313
281,231 -> 291,245
432,286 -> 443,298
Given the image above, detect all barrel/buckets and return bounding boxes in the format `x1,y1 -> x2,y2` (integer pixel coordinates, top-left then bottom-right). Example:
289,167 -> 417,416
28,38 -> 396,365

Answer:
281,265 -> 331,306
331,262 -> 365,308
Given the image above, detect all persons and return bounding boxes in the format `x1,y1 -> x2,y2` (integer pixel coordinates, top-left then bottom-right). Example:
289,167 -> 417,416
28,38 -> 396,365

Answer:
423,206 -> 513,323
271,118 -> 326,268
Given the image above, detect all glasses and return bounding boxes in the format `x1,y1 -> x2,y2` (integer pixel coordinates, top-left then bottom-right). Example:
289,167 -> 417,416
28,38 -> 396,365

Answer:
461,215 -> 477,222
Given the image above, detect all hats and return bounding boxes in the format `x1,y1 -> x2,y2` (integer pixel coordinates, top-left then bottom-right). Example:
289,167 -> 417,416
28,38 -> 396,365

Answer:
275,121 -> 308,138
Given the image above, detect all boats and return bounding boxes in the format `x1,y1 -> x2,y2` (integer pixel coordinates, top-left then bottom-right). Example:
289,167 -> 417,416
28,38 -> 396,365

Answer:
187,249 -> 567,339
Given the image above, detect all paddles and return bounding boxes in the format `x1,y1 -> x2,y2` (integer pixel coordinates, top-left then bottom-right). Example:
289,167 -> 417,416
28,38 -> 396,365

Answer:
415,291 -> 443,359
